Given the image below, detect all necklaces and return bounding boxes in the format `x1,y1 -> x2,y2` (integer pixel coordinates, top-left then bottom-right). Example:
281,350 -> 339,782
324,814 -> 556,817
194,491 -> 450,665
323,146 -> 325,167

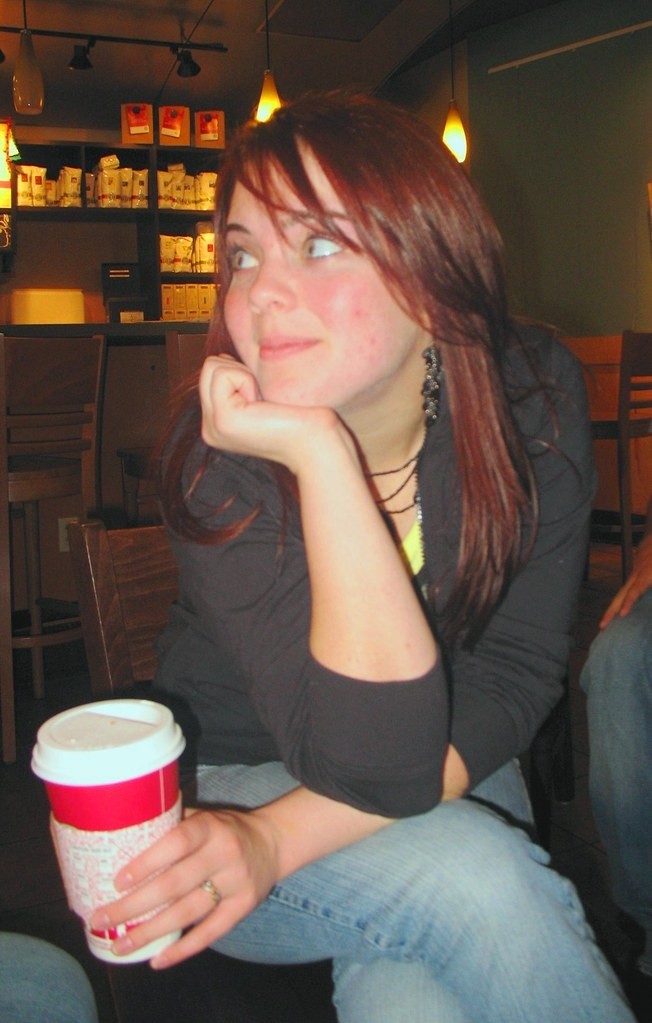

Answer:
366,457 -> 418,514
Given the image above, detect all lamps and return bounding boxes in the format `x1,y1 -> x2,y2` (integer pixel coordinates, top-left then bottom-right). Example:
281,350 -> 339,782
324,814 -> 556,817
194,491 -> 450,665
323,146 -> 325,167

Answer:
256,0 -> 281,123
177,49 -> 200,77
65,44 -> 92,71
12,0 -> 45,114
441,0 -> 467,164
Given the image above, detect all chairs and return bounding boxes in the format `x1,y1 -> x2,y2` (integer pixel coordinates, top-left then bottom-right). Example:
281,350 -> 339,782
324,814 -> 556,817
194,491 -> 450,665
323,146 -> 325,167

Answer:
70,520 -> 181,698
584,330 -> 652,582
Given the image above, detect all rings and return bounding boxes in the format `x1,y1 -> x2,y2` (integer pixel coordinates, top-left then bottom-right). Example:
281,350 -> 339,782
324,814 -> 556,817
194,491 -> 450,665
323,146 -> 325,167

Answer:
203,881 -> 222,905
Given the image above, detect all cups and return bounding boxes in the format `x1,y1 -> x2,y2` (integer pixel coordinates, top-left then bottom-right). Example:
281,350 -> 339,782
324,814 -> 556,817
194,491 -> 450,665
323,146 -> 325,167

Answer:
30,698 -> 185,964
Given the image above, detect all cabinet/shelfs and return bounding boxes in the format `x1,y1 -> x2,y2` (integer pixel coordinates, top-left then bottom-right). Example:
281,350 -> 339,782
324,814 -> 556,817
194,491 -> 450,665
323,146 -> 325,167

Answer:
0,140 -> 224,320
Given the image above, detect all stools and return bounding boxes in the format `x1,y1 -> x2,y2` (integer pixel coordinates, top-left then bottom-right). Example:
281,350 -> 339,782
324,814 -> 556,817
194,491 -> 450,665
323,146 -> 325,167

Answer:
102,331 -> 210,529
0,334 -> 104,768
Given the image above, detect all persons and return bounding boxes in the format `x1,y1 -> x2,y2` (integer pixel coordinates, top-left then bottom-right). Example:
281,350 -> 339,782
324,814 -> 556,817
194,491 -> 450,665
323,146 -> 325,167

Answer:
90,90 -> 640,1023
579,531 -> 652,1023
